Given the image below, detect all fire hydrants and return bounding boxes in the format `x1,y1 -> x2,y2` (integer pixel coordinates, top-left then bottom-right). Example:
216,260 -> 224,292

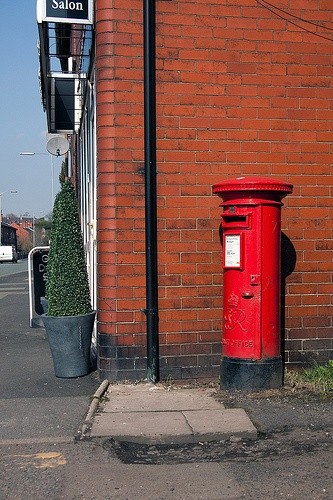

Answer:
213,176 -> 294,393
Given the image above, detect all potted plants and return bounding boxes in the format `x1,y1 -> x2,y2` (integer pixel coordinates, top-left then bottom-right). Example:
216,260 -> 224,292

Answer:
38,190 -> 96,378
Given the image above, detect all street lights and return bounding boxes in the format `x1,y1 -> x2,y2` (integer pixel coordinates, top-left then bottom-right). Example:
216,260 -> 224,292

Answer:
20,152 -> 55,212
0,190 -> 18,220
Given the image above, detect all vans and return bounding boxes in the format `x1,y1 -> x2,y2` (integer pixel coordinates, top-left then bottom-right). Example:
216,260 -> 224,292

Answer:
0,245 -> 18,263
